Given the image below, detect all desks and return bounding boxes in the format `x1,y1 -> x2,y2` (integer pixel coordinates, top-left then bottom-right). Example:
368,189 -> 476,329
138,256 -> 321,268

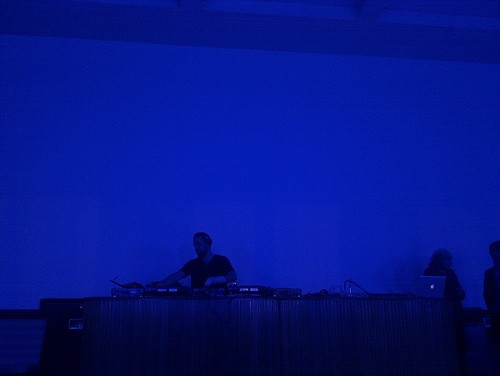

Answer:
41,294 -> 462,376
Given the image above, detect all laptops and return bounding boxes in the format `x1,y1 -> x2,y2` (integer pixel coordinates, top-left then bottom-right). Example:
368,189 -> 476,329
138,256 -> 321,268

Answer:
415,275 -> 447,298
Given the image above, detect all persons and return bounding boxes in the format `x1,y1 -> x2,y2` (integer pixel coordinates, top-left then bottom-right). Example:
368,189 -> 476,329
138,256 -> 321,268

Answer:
423,251 -> 470,376
483,241 -> 500,338
144,231 -> 237,289
